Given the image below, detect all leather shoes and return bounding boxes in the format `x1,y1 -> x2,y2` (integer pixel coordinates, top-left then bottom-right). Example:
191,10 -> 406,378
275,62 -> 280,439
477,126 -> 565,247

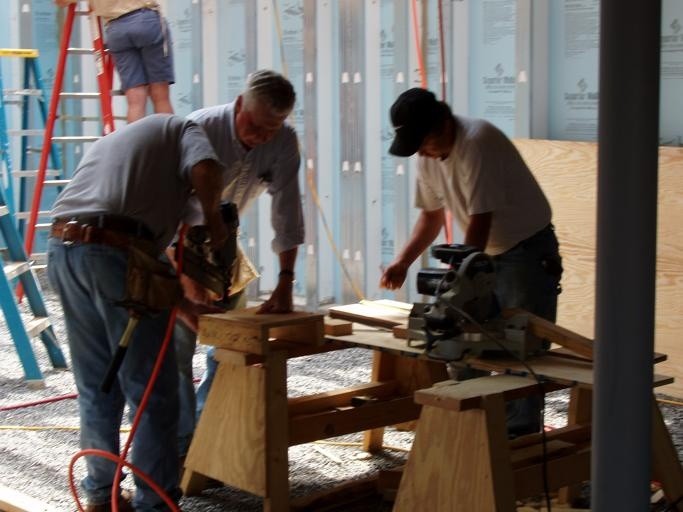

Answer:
86,494 -> 134,511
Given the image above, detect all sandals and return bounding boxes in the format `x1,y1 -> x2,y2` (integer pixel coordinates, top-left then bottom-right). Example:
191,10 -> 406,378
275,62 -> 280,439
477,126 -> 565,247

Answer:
125,247 -> 178,309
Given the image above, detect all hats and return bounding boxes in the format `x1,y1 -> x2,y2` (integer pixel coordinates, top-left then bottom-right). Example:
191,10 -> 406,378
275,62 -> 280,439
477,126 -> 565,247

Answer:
388,88 -> 435,157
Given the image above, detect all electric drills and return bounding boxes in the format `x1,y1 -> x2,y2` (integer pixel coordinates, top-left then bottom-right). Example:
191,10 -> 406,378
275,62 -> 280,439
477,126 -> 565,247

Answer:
186,200 -> 239,310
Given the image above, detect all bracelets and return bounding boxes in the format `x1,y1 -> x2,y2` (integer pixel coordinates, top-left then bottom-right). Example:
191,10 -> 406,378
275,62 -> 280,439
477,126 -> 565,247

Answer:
278,268 -> 296,284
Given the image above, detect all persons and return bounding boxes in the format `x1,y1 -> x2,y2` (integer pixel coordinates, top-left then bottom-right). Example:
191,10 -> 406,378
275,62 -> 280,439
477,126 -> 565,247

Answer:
172,67 -> 309,489
45,114 -> 233,511
86,0 -> 176,127
379,85 -> 564,437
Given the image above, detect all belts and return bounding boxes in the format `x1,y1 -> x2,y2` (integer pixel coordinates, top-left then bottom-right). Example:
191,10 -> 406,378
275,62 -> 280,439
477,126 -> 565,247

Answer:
51,220 -> 151,252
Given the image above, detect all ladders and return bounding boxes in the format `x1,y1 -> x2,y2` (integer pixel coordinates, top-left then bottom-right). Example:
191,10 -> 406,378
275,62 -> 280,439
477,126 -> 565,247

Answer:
1,49 -> 69,226
1,185 -> 67,390
12,0 -> 127,305
